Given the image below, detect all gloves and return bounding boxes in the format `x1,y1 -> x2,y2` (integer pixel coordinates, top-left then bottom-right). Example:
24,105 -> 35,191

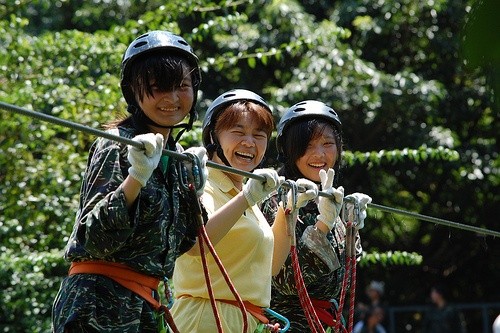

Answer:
243,168 -> 285,207
182,146 -> 208,198
279,179 -> 318,210
317,168 -> 344,230
127,133 -> 163,186
343,193 -> 372,230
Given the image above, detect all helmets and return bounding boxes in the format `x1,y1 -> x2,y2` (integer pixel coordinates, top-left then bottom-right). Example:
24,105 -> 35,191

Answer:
277,100 -> 342,191
202,89 -> 273,184
120,31 -> 202,147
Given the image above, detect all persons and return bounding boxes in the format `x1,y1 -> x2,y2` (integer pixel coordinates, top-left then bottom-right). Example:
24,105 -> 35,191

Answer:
51,30 -> 210,333
257,99 -> 372,333
354,279 -> 500,333
166,89 -> 319,333
